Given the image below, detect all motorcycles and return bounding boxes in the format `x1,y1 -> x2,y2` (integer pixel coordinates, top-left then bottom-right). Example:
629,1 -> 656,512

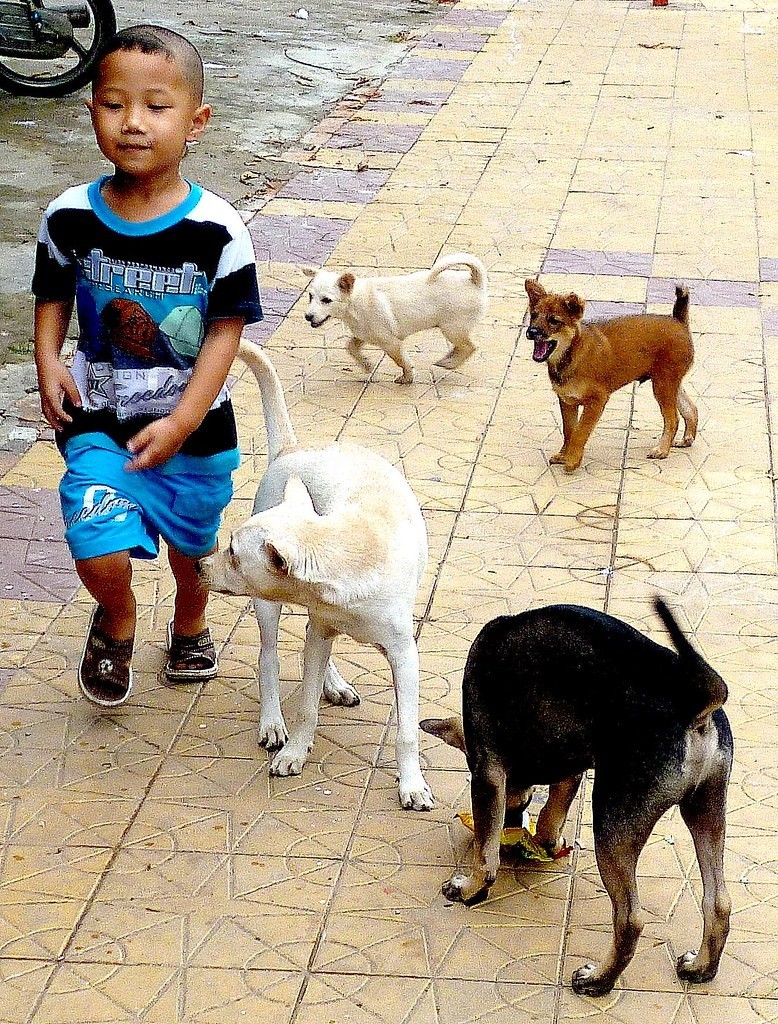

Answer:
0,0 -> 117,96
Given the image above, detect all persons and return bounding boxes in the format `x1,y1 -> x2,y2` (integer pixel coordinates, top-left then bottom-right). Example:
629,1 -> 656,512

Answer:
31,23 -> 263,705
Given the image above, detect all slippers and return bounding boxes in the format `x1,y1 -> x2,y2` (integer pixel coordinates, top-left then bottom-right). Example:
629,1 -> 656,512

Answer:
164,617 -> 220,680
76,602 -> 138,709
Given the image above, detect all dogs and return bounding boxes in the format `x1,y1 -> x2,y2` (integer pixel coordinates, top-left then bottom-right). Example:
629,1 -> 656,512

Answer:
302,252 -> 487,384
525,278 -> 699,472
417,594 -> 735,997
195,339 -> 435,811
